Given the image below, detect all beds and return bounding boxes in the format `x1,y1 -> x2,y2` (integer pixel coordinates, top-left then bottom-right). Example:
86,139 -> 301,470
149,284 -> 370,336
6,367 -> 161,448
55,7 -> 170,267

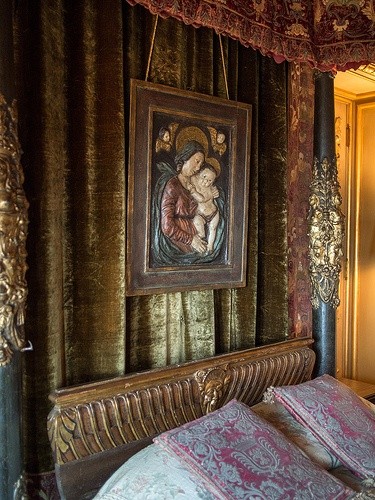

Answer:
49,336 -> 374,500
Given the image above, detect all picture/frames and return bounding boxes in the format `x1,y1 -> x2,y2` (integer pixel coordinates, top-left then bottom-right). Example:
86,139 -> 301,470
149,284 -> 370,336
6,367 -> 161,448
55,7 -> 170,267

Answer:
126,78 -> 252,296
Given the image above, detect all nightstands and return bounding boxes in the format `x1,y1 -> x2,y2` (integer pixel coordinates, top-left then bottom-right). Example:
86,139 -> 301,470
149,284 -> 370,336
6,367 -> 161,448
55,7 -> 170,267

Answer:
334,377 -> 374,402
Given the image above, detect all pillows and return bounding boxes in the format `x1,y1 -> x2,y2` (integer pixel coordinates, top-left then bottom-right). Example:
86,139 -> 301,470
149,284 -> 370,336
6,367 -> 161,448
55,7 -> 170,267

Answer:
151,398 -> 357,500
267,375 -> 375,484
